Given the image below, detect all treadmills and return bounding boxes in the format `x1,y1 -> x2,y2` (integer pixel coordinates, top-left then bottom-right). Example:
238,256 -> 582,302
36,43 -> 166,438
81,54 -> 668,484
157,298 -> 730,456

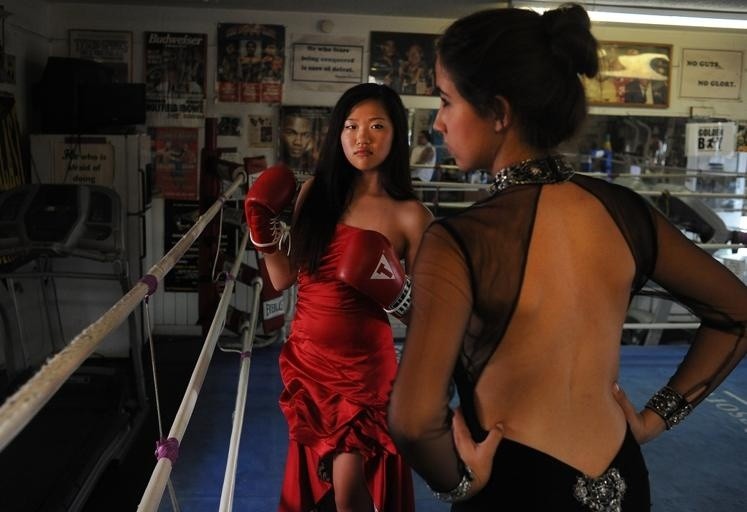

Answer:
0,183 -> 149,511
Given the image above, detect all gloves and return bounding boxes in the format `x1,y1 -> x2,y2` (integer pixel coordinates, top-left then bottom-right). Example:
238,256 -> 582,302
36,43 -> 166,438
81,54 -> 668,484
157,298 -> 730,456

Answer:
336,230 -> 415,319
246,163 -> 295,253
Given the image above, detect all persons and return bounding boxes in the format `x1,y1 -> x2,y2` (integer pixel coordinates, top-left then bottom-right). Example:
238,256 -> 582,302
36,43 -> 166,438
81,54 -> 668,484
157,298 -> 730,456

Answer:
444,159 -> 463,182
282,111 -> 319,174
148,139 -> 196,186
221,36 -> 281,84
399,43 -> 436,95
405,127 -> 435,183
388,3 -> 746,512
581,46 -> 668,106
371,37 -> 403,86
248,85 -> 435,512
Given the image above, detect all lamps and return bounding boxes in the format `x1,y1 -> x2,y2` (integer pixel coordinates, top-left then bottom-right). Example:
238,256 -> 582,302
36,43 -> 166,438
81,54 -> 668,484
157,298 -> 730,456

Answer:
508,0 -> 747,33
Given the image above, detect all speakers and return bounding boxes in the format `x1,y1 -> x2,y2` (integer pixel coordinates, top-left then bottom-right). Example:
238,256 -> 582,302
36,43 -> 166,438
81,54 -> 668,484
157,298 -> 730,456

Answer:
41,57 -> 111,134
